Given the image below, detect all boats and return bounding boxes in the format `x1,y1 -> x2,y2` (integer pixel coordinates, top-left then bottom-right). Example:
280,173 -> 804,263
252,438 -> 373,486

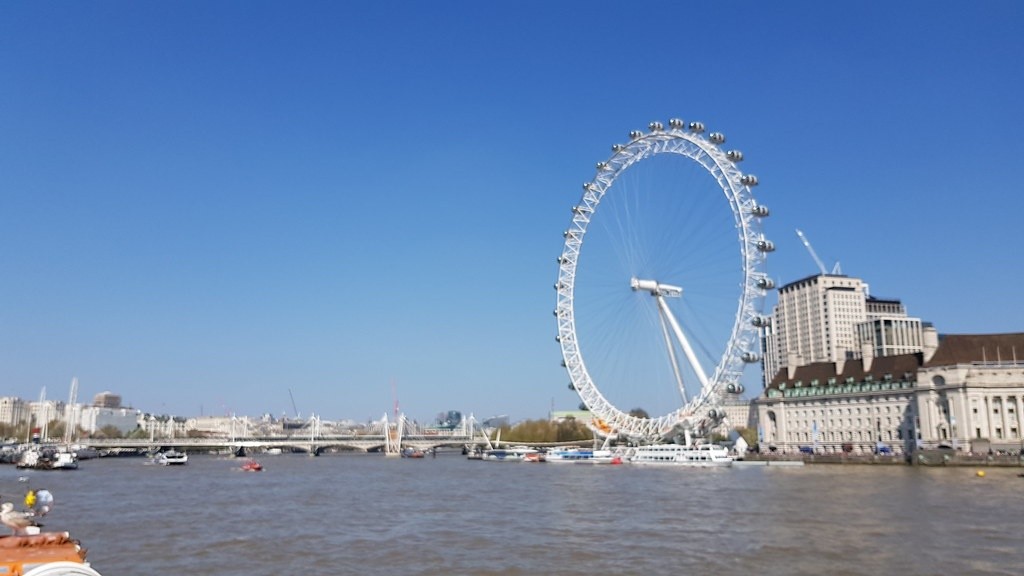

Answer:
542,451 -> 622,465
468,451 -> 482,460
401,449 -> 425,458
482,450 -> 540,462
160,450 -> 187,465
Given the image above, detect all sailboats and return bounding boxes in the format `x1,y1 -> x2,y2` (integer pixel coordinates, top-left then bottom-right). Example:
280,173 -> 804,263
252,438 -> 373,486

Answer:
0,376 -> 102,470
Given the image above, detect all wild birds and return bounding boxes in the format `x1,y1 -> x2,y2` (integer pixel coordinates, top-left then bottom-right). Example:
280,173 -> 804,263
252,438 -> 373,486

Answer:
0,502 -> 34,536
